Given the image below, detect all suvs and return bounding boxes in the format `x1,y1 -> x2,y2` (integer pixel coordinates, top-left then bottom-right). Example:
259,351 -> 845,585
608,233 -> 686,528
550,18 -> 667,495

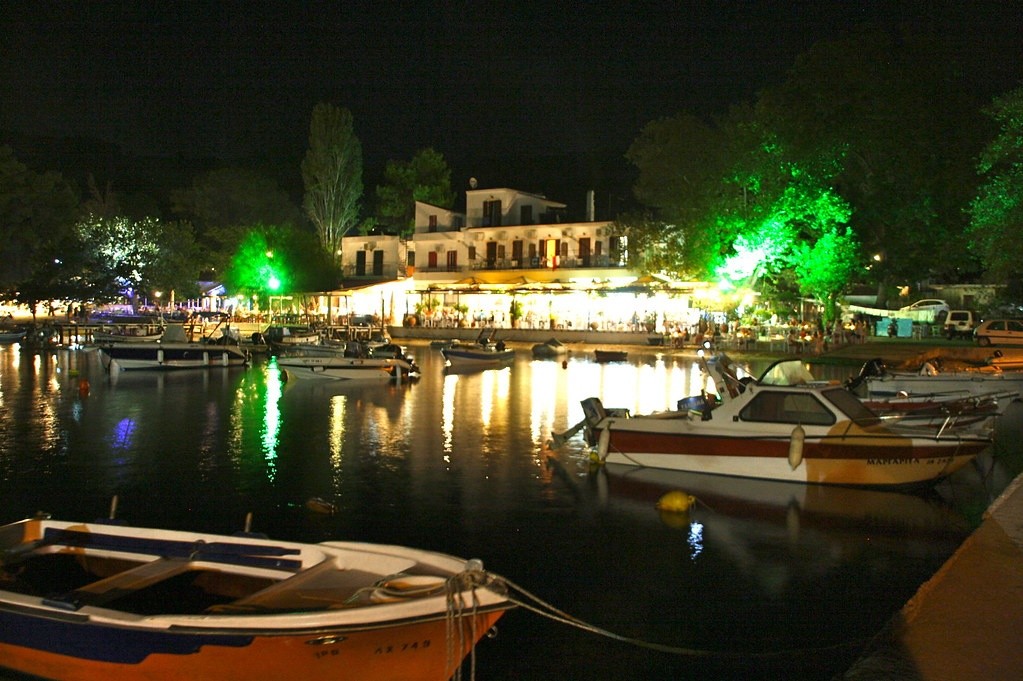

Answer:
898,298 -> 950,323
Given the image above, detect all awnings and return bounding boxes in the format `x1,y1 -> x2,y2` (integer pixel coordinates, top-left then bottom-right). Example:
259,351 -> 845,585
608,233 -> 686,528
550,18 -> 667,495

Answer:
312,278 -> 394,292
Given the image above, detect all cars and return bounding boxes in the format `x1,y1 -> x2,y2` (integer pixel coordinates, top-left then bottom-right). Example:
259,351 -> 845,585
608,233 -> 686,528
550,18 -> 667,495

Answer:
0,328 -> 29,344
971,318 -> 1023,347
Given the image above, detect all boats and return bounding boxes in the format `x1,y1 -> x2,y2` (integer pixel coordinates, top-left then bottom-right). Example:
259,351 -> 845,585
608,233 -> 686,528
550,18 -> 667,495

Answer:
431,340 -> 449,350
438,333 -> 515,366
551,355 -> 1023,488
0,517 -> 523,681
595,350 -> 628,363
90,312 -> 421,383
531,339 -> 567,358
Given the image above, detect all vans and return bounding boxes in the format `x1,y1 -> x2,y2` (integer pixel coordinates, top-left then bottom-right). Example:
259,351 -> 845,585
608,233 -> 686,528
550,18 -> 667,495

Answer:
943,309 -> 981,332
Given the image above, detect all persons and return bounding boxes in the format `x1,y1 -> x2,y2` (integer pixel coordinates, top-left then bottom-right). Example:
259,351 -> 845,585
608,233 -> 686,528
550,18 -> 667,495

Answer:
524,311 -> 545,329
789,316 -> 867,355
155,304 -> 159,312
663,311 -> 759,350
473,309 -> 505,325
631,310 -> 650,330
700,388 -> 717,421
273,301 -> 314,314
48,301 -> 55,316
68,302 -> 85,321
176,304 -> 196,312
228,301 -> 259,313
8,312 -> 12,318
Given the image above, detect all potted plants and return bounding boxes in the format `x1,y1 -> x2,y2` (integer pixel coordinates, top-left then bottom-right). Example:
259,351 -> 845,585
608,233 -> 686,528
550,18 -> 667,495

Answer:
509,300 -> 525,328
644,310 -> 658,332
454,304 -> 469,327
548,312 -> 559,328
590,311 -> 604,330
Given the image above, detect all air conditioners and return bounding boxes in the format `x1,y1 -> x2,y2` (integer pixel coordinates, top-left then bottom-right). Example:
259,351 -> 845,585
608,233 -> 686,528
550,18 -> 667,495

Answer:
527,231 -> 537,239
562,230 -> 572,238
363,243 -> 372,250
473,232 -> 483,240
595,228 -> 606,236
435,246 -> 445,253
497,232 -> 507,240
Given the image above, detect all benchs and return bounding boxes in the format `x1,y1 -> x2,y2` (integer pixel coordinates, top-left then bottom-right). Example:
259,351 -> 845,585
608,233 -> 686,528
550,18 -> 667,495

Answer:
73,557 -> 187,604
